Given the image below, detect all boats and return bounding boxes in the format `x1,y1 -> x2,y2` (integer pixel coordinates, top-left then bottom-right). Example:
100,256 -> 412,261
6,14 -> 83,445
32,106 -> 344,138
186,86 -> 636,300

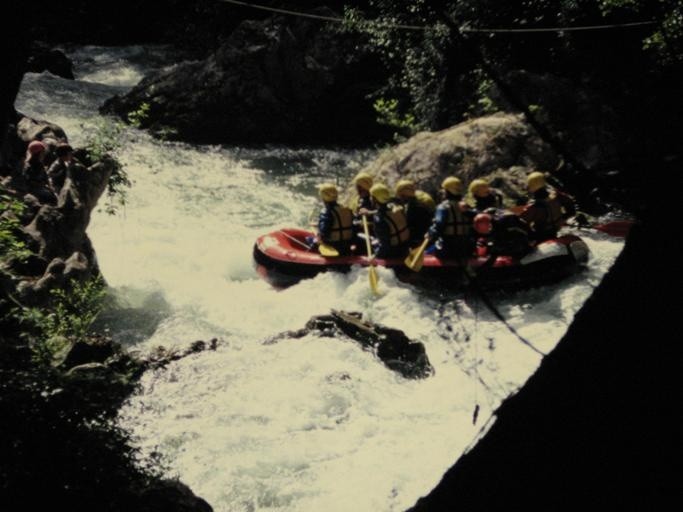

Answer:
251,226 -> 590,291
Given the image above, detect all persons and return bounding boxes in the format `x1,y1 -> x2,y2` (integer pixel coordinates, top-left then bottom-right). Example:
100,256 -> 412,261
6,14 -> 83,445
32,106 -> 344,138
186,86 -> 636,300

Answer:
306,171 -> 576,268
20,140 -> 74,206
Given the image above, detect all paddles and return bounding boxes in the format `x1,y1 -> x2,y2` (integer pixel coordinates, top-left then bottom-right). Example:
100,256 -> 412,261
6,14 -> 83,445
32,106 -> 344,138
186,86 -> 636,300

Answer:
309,223 -> 339,257
404,239 -> 429,271
563,222 -> 632,235
363,214 -> 386,296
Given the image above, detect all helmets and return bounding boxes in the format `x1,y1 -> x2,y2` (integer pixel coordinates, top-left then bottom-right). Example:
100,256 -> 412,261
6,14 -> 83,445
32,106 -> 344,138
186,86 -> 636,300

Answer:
474,214 -> 491,234
396,181 -> 415,197
441,177 -> 463,196
470,181 -> 489,197
369,182 -> 390,204
354,172 -> 373,190
319,184 -> 338,201
526,172 -> 545,194
